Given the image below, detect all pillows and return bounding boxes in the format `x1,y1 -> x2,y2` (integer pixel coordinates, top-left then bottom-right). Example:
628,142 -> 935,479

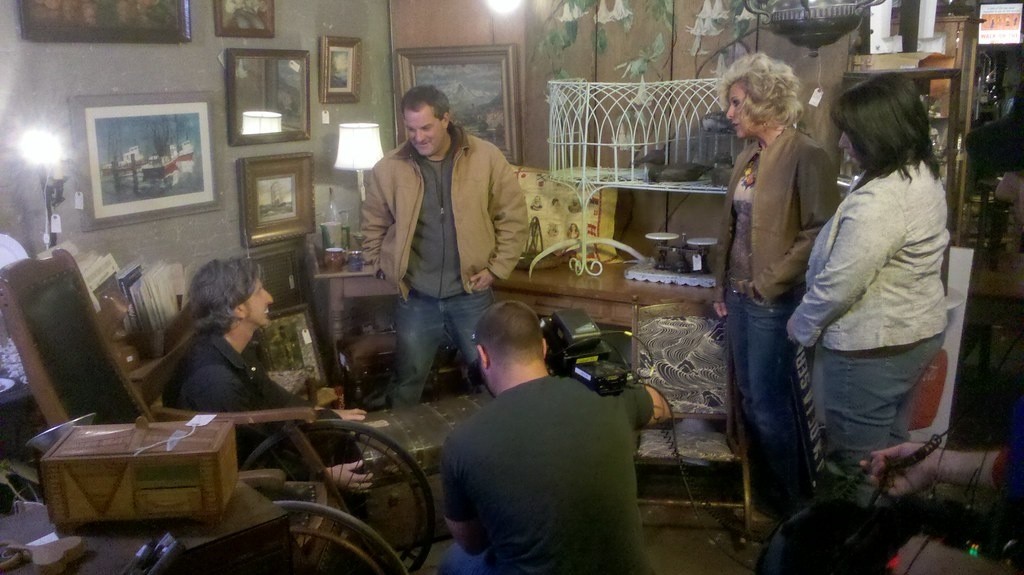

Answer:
508,164 -> 625,263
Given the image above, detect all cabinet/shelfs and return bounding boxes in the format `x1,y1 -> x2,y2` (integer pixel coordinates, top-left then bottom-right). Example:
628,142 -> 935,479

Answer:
841,68 -> 963,231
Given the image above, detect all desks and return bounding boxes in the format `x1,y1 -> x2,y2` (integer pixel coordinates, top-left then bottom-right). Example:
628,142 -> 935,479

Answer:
494,254 -> 718,338
307,242 -> 476,402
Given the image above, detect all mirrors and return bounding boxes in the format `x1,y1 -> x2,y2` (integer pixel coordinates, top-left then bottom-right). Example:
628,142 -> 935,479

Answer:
224,46 -> 313,146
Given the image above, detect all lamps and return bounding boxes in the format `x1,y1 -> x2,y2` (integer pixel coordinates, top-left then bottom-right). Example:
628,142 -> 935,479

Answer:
240,109 -> 285,136
18,130 -> 72,246
330,121 -> 386,198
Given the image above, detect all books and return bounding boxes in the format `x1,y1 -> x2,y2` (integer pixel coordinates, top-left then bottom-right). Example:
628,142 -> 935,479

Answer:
38,240 -> 183,332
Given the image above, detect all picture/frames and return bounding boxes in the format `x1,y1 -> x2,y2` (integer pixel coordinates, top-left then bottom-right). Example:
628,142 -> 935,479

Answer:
393,42 -> 524,165
318,31 -> 364,106
67,91 -> 225,228
211,0 -> 276,40
258,298 -> 328,387
18,0 -> 193,45
235,151 -> 319,249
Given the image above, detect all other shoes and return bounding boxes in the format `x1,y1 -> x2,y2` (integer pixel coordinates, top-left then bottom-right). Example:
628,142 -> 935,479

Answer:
734,502 -> 782,528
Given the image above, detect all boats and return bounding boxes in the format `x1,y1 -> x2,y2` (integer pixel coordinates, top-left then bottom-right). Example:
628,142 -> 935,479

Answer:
99,144 -> 145,176
138,132 -> 194,191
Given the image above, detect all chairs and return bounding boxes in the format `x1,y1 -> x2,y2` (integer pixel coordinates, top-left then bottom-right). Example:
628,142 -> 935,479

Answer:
625,290 -> 755,552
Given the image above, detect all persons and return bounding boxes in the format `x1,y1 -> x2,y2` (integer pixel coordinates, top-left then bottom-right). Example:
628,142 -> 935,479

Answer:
861,82 -> 1024,575
439,299 -> 672,575
713,52 -> 842,528
785,72 -> 950,508
160,259 -> 373,526
360,86 -> 529,410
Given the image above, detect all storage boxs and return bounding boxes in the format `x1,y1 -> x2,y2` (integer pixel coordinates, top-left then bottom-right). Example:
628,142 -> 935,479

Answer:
132,300 -> 192,357
354,388 -> 492,554
38,415 -> 241,535
